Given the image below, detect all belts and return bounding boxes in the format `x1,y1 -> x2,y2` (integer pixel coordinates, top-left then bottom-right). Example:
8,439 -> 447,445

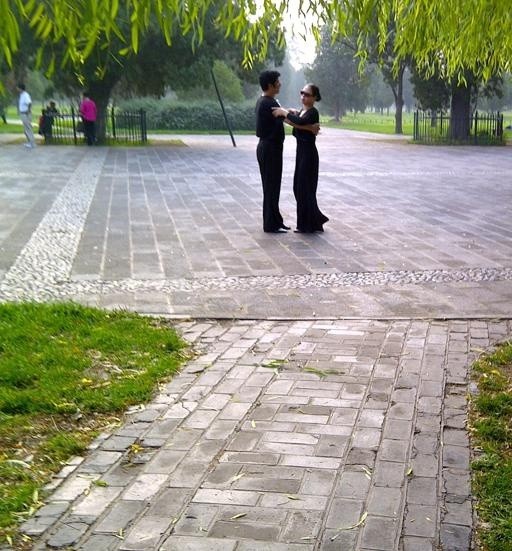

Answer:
21,112 -> 27,114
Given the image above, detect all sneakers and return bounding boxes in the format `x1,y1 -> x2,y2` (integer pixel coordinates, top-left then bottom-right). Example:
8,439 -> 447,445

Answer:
23,143 -> 34,148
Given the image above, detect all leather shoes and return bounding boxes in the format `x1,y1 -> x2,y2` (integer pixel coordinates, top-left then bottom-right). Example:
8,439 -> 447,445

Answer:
293,229 -> 310,233
276,224 -> 290,233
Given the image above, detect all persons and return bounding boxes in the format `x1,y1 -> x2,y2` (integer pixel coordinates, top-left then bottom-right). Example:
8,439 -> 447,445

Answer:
256,71 -> 329,233
16,81 -> 37,148
80,92 -> 98,145
39,101 -> 63,144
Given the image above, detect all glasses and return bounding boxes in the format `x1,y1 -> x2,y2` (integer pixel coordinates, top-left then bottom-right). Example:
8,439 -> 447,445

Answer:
300,91 -> 315,98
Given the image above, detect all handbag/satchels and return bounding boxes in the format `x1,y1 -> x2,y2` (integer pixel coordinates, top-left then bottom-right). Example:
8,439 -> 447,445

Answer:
76,122 -> 91,133
